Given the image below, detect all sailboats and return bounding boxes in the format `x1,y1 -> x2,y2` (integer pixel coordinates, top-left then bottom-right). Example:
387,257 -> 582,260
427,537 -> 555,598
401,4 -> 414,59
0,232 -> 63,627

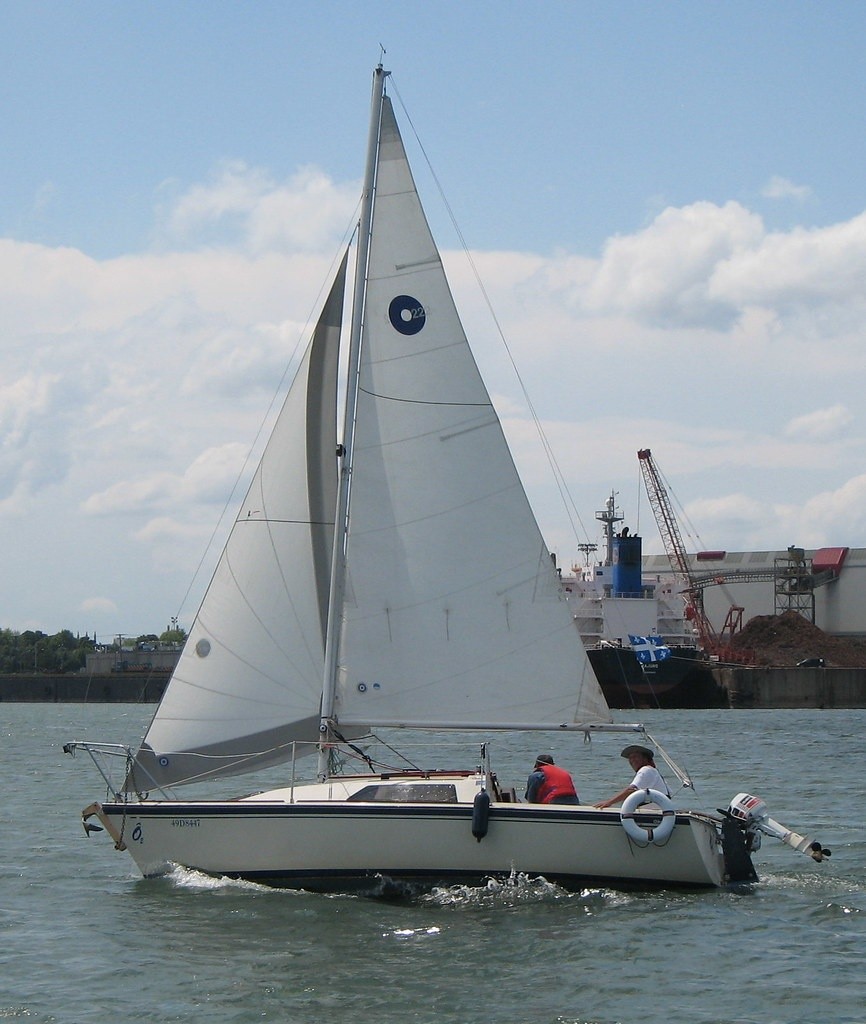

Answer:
62,45 -> 832,890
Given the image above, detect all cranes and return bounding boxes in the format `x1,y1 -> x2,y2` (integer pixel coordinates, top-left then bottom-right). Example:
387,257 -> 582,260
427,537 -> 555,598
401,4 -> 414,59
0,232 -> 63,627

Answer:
635,448 -> 756,666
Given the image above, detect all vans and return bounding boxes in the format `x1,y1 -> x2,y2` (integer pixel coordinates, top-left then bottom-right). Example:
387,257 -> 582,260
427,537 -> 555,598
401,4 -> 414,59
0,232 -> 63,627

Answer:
796,658 -> 825,668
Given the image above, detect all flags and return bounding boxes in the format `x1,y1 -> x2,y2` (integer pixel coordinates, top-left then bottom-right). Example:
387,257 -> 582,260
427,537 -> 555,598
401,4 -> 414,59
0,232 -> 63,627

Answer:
628,634 -> 671,663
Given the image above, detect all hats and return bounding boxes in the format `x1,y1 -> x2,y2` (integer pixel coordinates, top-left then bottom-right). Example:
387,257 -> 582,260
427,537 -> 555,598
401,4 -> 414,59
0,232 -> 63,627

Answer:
534,755 -> 554,768
621,745 -> 653,758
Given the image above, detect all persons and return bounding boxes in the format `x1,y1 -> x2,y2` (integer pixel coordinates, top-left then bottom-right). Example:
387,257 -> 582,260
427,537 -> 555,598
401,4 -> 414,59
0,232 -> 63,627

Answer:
594,745 -> 670,811
524,755 -> 581,806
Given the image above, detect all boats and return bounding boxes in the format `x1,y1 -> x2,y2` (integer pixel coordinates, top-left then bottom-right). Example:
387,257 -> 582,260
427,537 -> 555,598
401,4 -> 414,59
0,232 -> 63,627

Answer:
544,490 -> 710,711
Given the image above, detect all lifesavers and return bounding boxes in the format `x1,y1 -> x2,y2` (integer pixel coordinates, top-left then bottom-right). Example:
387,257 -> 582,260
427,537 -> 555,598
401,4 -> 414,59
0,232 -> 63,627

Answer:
620,788 -> 676,842
